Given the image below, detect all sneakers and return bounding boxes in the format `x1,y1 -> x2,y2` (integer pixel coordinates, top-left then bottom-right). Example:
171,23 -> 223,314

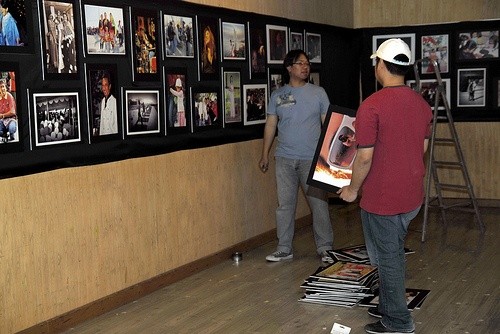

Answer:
266,249 -> 293,261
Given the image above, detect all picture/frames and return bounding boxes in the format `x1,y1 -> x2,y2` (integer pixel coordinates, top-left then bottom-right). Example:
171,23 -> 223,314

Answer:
0,0 -> 356,195
373,34 -> 415,68
298,243 -> 430,310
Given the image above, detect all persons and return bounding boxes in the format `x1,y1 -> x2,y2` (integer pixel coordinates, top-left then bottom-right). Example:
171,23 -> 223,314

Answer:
0,3 -> 321,143
422,32 -> 498,106
336,38 -> 434,334
259,50 -> 334,262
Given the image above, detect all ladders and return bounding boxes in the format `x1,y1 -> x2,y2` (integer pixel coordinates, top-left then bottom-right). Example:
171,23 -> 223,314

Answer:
413,57 -> 485,243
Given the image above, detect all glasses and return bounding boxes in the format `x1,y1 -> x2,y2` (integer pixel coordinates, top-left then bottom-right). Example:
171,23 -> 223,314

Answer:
292,61 -> 309,65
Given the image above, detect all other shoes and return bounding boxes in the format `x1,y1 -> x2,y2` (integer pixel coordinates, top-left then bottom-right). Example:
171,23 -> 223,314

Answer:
365,306 -> 414,334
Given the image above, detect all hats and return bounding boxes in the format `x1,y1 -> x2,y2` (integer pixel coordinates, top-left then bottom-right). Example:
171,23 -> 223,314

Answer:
175,78 -> 182,87
370,38 -> 412,66
